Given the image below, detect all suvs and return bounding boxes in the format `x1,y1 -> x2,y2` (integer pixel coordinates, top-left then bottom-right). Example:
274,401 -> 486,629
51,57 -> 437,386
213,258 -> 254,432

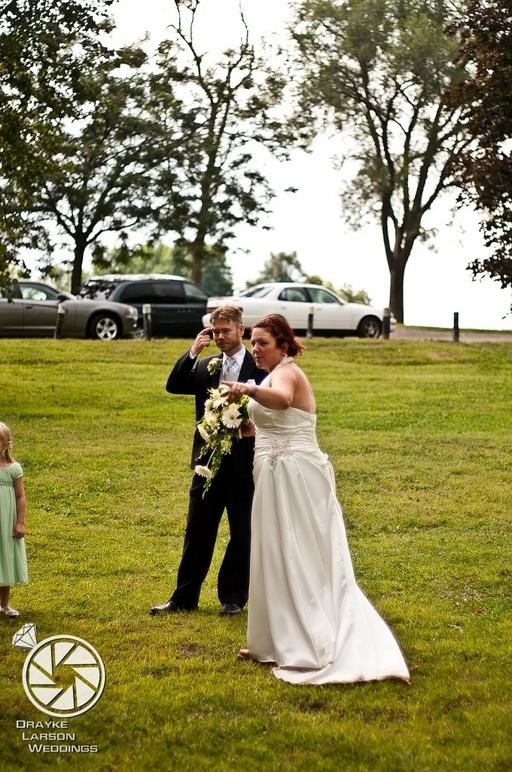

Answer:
80,275 -> 215,339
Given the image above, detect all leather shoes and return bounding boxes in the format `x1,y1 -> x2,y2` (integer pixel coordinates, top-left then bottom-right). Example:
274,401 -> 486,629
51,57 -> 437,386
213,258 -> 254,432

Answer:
239,648 -> 250,657
220,604 -> 241,616
149,600 -> 197,615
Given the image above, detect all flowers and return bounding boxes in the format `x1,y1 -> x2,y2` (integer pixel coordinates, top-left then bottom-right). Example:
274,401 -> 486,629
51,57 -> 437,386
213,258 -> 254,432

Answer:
192,383 -> 250,503
206,357 -> 224,376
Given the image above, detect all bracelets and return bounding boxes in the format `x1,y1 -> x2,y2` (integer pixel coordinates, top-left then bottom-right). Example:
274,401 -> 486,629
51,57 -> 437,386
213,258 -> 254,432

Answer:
249,387 -> 258,398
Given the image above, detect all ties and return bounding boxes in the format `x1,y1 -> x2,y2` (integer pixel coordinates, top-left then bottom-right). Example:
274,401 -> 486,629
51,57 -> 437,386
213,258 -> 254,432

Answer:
222,357 -> 236,381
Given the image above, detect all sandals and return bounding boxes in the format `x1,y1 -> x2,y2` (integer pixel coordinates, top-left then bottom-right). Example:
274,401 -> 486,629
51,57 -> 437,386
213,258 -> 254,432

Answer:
0,606 -> 20,616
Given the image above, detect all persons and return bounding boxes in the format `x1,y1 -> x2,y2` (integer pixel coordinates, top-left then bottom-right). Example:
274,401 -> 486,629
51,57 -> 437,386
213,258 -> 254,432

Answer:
219,312 -> 413,688
0,419 -> 30,618
145,303 -> 271,616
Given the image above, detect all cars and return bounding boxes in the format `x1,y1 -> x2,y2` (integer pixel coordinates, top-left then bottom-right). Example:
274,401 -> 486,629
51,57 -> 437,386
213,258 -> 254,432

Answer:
0,278 -> 140,340
206,282 -> 397,338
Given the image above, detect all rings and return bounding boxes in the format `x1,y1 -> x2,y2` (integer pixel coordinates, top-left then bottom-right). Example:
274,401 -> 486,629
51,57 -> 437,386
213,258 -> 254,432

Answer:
236,387 -> 240,390
